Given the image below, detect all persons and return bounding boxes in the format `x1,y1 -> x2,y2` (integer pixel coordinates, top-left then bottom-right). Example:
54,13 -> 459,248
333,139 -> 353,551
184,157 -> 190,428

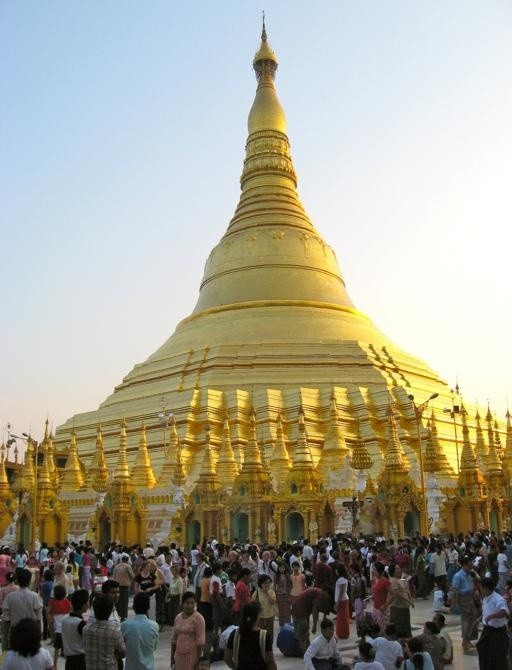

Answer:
60,589 -> 89,669
223,599 -> 277,670
169,590 -> 206,670
93,581 -> 125,667
1,529 -> 512,670
4,617 -> 53,670
3,568 -> 45,649
83,593 -> 126,670
303,618 -> 348,670
120,591 -> 161,670
475,576 -> 510,669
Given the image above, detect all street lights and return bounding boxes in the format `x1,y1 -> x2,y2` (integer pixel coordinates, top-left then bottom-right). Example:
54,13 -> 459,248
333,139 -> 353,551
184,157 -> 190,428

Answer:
446,402 -> 468,475
157,406 -> 175,469
344,492 -> 366,546
9,426 -> 42,553
404,389 -> 440,510
